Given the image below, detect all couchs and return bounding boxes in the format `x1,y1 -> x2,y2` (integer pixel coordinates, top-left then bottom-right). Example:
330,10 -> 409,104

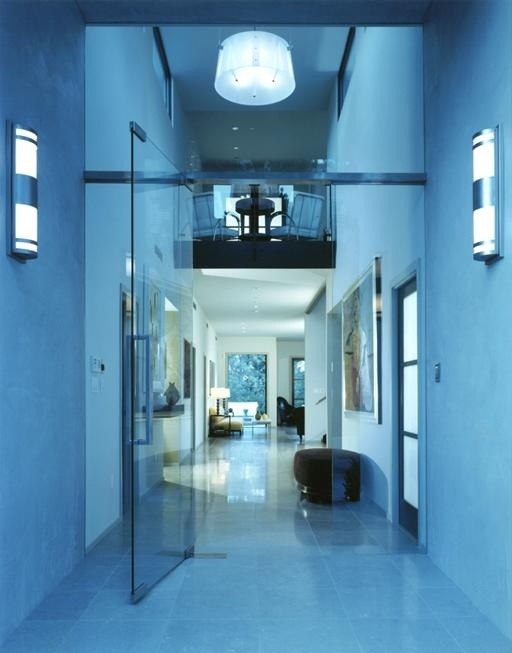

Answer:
210,407 -> 244,436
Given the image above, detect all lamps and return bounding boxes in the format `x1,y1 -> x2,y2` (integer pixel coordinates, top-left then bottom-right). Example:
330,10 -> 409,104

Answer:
210,387 -> 230,416
6,118 -> 40,263
214,24 -> 296,107
470,122 -> 504,267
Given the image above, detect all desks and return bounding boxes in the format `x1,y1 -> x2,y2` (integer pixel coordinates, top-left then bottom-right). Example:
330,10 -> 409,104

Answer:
251,417 -> 272,440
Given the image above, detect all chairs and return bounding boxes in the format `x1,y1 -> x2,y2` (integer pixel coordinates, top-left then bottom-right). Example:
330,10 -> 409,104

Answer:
193,191 -> 240,243
267,190 -> 326,242
295,406 -> 304,445
277,396 -> 295,426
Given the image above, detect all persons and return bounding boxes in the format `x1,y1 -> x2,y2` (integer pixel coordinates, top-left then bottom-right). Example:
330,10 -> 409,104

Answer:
349,286 -> 372,412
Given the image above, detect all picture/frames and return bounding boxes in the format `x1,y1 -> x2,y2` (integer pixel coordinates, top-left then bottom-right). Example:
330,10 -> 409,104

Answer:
341,257 -> 383,424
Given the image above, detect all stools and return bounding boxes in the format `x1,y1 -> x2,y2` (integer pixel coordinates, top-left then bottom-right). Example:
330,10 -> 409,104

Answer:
294,446 -> 361,504
